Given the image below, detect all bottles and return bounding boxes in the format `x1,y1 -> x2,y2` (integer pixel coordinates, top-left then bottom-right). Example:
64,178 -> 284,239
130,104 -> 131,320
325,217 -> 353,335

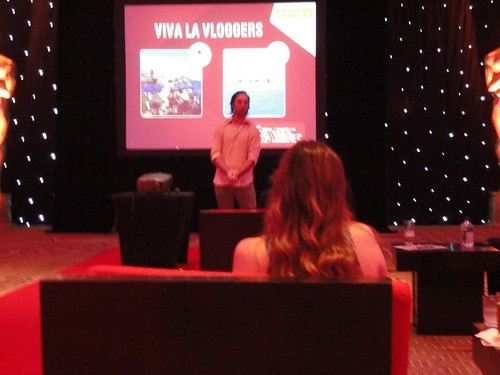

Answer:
460,216 -> 474,248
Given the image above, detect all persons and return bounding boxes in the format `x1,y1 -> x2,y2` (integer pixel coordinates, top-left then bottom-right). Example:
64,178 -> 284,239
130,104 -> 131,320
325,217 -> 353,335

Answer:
484,48 -> 500,163
210,91 -> 261,209
0,55 -> 17,167
231,139 -> 387,282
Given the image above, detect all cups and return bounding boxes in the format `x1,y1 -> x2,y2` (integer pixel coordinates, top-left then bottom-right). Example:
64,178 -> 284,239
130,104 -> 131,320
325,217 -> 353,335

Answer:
482,291 -> 500,329
403,219 -> 415,247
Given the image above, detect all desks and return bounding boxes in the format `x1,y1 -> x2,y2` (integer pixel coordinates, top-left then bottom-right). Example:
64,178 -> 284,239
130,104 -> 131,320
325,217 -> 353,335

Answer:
198,209 -> 266,272
112,192 -> 193,268
392,244 -> 499,335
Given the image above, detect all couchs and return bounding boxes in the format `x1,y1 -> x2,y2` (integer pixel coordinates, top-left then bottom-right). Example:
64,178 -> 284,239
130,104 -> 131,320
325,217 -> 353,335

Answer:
39,265 -> 411,375
